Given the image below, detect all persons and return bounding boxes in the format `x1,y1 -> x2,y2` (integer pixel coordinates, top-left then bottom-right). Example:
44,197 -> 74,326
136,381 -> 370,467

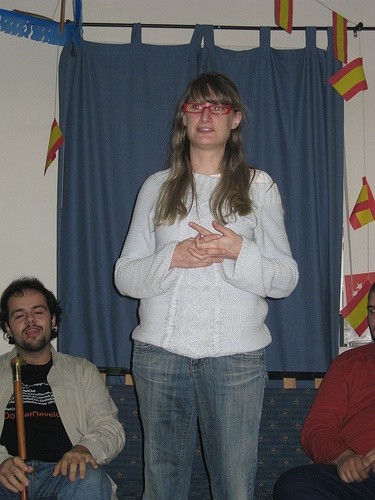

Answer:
112,71 -> 301,500
0,277 -> 125,500
272,281 -> 375,499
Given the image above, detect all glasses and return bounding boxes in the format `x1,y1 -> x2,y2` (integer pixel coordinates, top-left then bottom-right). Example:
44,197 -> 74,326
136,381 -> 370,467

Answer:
182,102 -> 236,115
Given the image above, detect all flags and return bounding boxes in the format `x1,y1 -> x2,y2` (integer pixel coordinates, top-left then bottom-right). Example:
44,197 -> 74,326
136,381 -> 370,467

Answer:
332,11 -> 349,65
328,57 -> 368,101
43,118 -> 64,176
59,1 -> 66,34
349,177 -> 375,230
339,282 -> 371,338
274,0 -> 294,34
